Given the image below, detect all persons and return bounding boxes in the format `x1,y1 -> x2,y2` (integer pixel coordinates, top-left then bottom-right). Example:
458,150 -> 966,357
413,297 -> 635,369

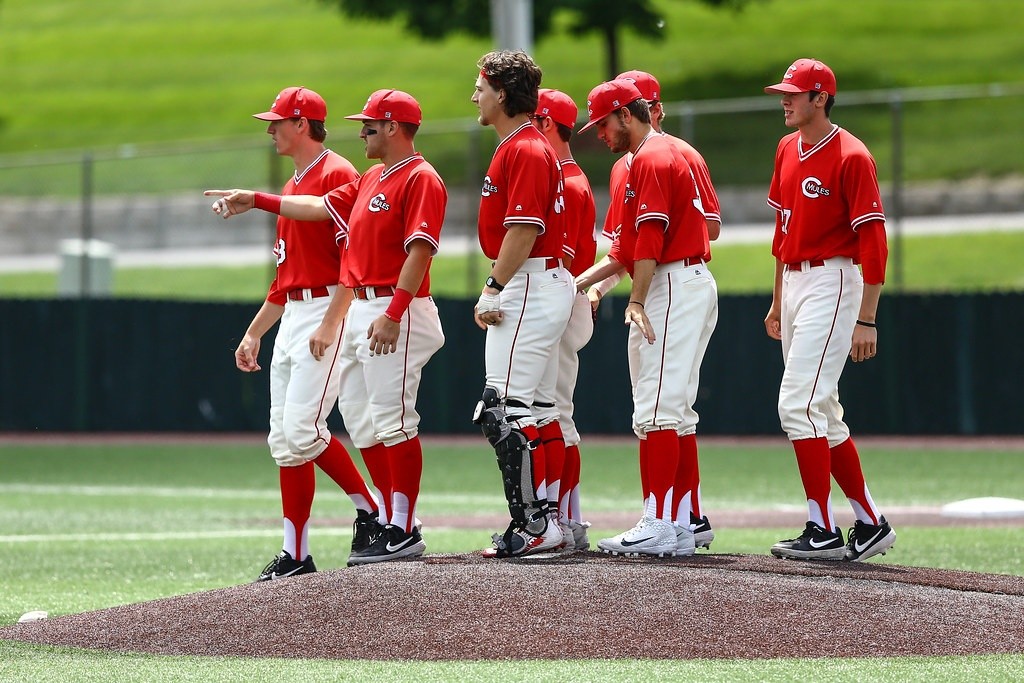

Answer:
235,86 -> 382,581
471,50 -> 596,561
763,59 -> 897,562
205,91 -> 446,566
575,70 -> 722,557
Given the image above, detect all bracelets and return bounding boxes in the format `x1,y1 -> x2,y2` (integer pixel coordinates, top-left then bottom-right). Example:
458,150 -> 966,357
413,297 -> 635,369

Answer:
594,273 -> 621,299
254,192 -> 282,215
384,288 -> 415,323
856,319 -> 877,328
628,301 -> 644,309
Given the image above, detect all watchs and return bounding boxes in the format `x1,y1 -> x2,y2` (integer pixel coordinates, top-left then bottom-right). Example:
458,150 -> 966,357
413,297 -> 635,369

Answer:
485,276 -> 505,292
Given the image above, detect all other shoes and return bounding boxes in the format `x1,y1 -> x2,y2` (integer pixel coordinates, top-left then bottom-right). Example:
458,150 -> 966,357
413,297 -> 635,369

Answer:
491,258 -> 559,271
289,287 -> 329,301
684,256 -> 701,266
786,260 -> 825,271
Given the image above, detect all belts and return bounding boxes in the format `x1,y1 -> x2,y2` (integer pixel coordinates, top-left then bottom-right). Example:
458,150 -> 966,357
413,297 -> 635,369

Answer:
355,286 -> 396,299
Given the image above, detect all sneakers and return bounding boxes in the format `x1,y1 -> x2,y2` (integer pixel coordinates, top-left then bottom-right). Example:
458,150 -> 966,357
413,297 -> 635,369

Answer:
257,550 -> 318,583
346,508 -> 426,567
690,511 -> 715,550
771,520 -> 847,559
597,513 -> 695,557
482,512 -> 591,557
843,515 -> 896,562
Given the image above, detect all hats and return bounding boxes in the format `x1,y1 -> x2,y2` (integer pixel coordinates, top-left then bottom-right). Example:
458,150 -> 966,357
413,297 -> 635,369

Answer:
252,85 -> 327,123
343,88 -> 422,126
534,88 -> 578,129
764,57 -> 836,96
576,79 -> 643,135
614,69 -> 660,103
